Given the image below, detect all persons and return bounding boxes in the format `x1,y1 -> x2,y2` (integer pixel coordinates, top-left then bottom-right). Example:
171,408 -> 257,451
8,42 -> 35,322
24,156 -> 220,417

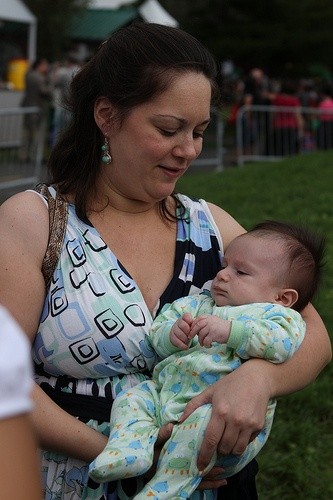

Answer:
88,220 -> 323,500
17,56 -> 332,168
0,21 -> 332,500
